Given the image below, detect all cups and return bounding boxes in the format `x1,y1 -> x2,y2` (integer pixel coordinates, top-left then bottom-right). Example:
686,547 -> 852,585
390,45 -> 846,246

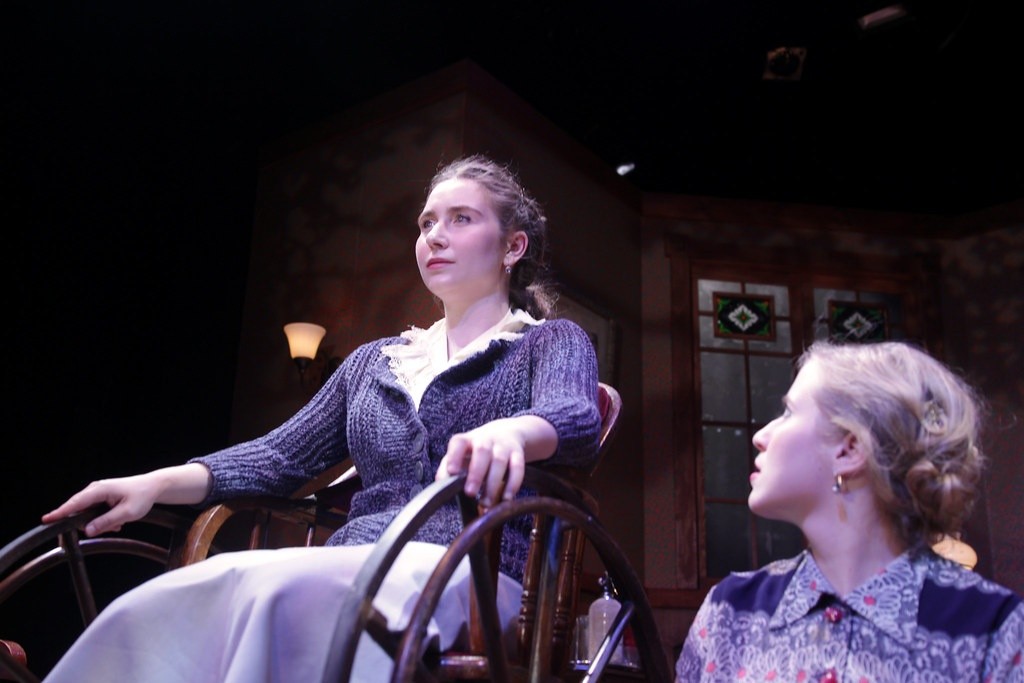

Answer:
572,614 -> 590,666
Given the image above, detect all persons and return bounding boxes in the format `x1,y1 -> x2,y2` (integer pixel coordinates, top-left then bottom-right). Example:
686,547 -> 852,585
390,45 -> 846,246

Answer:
41,159 -> 603,683
676,340 -> 1024,683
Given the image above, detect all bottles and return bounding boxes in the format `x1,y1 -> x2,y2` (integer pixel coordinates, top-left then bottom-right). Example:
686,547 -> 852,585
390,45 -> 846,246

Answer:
588,578 -> 624,663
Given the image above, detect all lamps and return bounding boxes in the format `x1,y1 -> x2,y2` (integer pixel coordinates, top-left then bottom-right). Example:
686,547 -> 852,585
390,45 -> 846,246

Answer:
284,323 -> 344,395
